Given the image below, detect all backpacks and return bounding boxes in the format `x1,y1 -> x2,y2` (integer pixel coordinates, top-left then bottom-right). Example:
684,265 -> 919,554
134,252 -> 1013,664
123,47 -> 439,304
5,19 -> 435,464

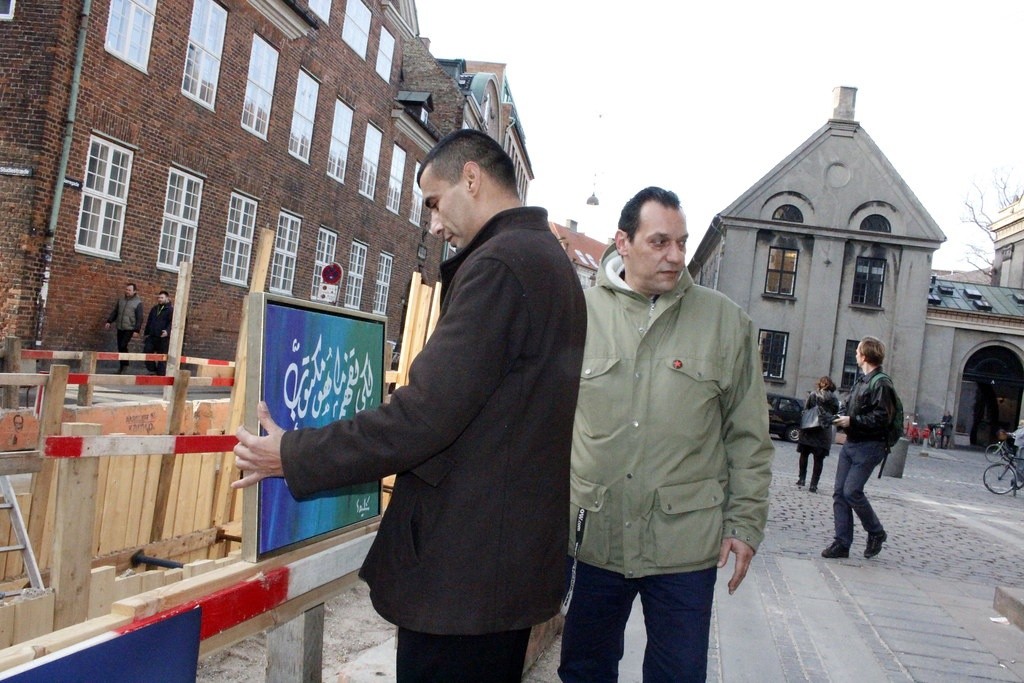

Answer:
870,373 -> 904,449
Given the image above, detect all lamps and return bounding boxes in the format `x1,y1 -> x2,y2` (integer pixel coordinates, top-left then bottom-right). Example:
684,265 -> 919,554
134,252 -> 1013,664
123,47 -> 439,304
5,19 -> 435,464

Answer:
587,192 -> 600,205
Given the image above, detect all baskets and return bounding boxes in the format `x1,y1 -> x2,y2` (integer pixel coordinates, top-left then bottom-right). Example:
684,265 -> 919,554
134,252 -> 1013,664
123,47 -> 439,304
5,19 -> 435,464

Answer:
999,432 -> 1007,440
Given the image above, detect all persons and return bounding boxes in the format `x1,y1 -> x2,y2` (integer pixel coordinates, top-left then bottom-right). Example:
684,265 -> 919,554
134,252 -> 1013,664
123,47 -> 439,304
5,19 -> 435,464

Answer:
557,186 -> 775,683
143,291 -> 174,375
796,376 -> 839,493
105,283 -> 143,373
820,336 -> 898,558
230,129 -> 588,683
941,411 -> 953,449
1012,420 -> 1024,454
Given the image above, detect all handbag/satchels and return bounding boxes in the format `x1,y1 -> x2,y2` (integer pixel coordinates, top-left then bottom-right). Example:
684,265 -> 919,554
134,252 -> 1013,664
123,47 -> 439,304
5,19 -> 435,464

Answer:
800,393 -> 824,429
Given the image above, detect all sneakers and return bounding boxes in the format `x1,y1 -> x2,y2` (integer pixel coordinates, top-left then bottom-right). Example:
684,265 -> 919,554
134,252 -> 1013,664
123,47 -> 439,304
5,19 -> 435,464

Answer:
822,538 -> 848,558
863,530 -> 887,558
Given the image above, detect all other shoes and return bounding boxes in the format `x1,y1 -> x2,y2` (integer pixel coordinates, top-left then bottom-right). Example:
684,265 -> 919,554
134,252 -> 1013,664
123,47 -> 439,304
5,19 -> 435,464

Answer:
809,485 -> 817,492
119,366 -> 127,374
797,479 -> 805,485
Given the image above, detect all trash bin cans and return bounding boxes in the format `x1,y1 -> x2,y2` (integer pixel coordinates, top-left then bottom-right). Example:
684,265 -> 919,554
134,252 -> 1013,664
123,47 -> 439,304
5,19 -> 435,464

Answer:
881,437 -> 910,479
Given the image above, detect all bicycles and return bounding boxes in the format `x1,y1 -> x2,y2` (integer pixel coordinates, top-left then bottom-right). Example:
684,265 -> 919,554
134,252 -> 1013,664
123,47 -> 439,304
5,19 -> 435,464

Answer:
928,422 -> 942,450
985,430 -> 1015,463
903,413 -> 921,446
982,450 -> 1024,495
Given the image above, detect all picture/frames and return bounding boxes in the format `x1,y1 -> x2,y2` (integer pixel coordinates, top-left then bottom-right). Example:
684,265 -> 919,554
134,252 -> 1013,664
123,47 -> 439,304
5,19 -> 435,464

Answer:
242,290 -> 389,564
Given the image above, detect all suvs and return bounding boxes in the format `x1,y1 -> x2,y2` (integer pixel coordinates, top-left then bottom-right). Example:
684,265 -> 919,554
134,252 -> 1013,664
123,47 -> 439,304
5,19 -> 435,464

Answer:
767,392 -> 806,443
